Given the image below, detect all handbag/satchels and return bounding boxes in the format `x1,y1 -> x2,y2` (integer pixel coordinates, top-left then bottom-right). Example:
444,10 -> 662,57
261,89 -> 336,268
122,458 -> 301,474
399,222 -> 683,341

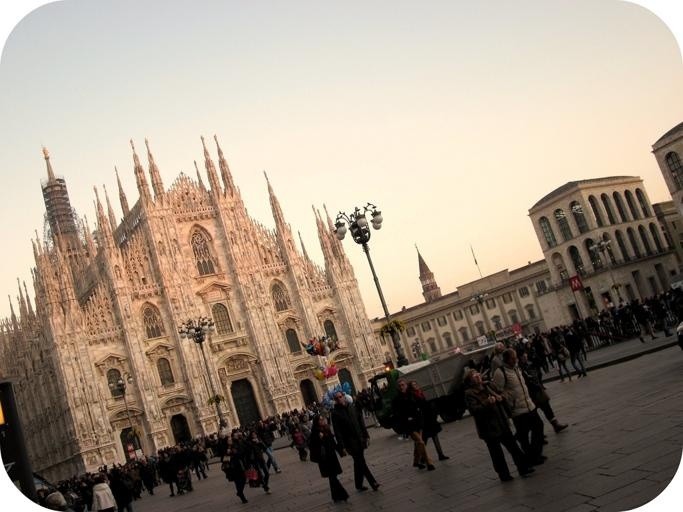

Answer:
309,446 -> 328,464
222,455 -> 231,470
246,463 -> 260,487
557,346 -> 570,361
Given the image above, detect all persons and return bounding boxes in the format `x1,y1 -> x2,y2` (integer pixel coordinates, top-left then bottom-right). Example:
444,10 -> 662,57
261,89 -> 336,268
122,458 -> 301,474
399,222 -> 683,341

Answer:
490,287 -> 683,383
34,384 -> 387,511
492,348 -> 548,466
394,378 -> 450,471
462,366 -> 536,481
516,346 -> 568,433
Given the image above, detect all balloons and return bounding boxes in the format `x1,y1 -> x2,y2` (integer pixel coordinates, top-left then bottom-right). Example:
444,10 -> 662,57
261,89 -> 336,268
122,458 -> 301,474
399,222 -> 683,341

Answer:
300,334 -> 342,380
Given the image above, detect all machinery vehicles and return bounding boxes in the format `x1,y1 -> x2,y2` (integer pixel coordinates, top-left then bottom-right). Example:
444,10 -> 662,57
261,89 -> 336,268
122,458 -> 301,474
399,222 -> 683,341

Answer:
368,342 -> 498,435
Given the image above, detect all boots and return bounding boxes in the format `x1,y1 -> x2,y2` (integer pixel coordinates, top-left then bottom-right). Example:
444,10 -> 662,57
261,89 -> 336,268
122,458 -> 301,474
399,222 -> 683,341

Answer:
550,416 -> 568,433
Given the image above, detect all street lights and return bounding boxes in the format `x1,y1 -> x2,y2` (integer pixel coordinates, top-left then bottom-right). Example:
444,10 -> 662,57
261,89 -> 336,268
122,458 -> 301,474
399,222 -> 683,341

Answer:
106,371 -> 144,460
470,293 -> 492,332
589,238 -> 625,306
333,202 -> 411,367
177,315 -> 230,430
